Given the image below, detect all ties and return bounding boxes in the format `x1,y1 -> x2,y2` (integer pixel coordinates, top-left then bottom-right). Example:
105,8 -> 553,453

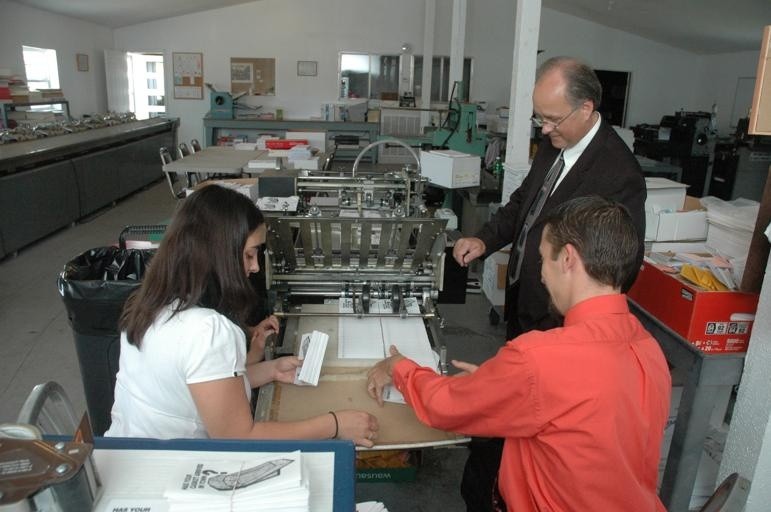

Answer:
510,152 -> 564,286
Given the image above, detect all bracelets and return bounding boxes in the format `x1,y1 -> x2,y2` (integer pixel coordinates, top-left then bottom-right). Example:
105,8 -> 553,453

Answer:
329,411 -> 338,439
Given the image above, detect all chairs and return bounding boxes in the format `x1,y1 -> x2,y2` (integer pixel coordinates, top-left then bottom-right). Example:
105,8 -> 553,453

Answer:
702,472 -> 750,511
159,139 -> 339,212
16,378 -> 91,512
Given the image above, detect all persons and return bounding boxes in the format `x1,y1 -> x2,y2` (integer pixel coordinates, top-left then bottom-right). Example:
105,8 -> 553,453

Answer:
103,184 -> 379,448
452,57 -> 647,512
244,314 -> 280,365
367,196 -> 672,512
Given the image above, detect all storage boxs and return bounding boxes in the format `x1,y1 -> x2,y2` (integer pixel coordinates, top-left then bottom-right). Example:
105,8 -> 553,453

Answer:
645,194 -> 709,242
627,249 -> 757,353
419,147 -> 482,192
482,251 -> 511,307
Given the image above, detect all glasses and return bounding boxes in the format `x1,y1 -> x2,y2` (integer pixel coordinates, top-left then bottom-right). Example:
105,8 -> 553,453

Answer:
531,109 -> 575,129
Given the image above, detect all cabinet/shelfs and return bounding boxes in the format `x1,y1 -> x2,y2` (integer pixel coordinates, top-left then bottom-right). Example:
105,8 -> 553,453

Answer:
202,117 -> 378,166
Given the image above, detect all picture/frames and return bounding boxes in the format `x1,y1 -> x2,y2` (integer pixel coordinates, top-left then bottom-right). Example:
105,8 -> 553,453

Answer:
76,52 -> 89,72
297,60 -> 317,77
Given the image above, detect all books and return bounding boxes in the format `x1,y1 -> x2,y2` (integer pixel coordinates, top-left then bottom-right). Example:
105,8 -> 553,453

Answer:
234,135 -> 320,170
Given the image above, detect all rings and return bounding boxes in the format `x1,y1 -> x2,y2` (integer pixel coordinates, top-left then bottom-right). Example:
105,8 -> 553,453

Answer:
368,431 -> 374,440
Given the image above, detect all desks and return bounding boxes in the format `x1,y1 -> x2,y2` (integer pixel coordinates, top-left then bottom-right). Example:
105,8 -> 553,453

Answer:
624,303 -> 747,512
